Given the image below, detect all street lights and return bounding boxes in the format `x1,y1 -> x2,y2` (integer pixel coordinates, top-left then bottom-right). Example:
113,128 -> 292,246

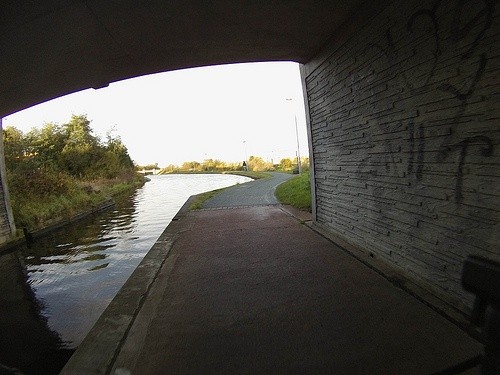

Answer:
286,98 -> 302,176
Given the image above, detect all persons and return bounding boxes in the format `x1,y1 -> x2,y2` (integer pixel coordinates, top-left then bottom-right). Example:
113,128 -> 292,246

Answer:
242,161 -> 247,173
